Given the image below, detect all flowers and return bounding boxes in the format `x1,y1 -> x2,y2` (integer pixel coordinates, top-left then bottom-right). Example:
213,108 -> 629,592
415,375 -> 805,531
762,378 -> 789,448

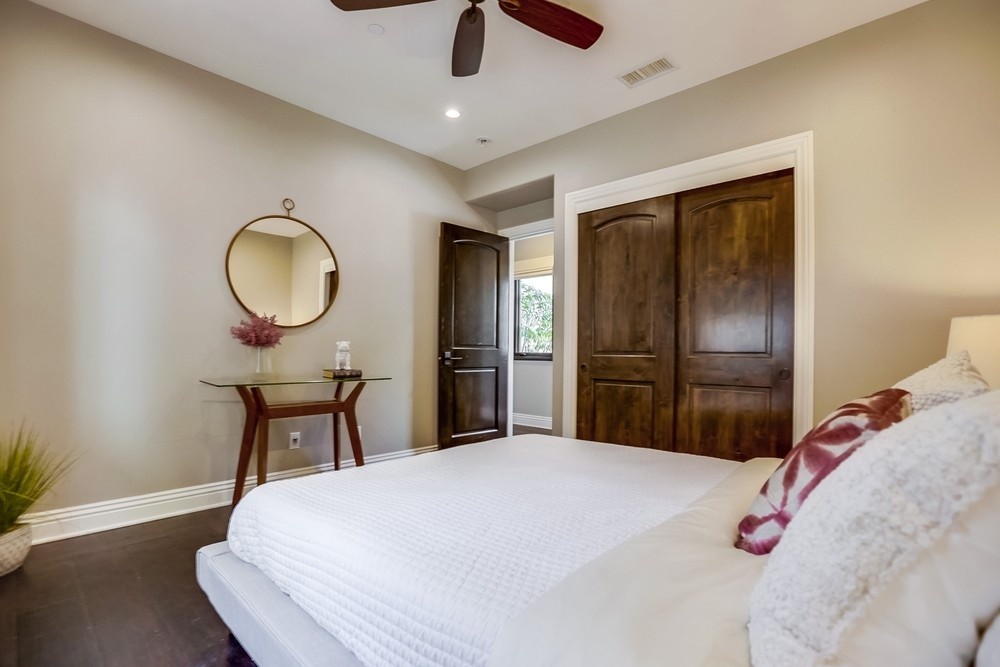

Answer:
227,310 -> 283,347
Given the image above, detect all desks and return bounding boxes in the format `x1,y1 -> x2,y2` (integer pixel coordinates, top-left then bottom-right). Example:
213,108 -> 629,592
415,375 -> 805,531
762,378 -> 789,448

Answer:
198,376 -> 394,510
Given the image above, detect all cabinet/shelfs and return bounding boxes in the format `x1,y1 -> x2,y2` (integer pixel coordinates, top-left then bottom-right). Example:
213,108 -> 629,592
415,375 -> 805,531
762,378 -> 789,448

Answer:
576,166 -> 794,464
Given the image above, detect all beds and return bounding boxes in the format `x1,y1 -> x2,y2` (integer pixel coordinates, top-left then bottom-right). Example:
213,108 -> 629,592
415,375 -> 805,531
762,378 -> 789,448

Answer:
196,435 -> 999,667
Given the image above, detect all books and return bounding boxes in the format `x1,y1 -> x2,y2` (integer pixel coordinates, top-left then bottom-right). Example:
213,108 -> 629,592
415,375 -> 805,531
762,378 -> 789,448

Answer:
323,369 -> 362,379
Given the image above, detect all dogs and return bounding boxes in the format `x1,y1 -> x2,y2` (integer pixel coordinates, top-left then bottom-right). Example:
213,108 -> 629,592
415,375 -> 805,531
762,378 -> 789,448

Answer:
335,341 -> 352,370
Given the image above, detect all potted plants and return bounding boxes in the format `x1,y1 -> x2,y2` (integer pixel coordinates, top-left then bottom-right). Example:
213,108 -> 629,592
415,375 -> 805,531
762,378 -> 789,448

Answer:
1,417 -> 91,579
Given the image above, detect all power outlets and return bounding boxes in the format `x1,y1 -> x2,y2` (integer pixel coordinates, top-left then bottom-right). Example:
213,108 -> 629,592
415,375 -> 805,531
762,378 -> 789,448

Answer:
357,425 -> 361,440
290,432 -> 301,449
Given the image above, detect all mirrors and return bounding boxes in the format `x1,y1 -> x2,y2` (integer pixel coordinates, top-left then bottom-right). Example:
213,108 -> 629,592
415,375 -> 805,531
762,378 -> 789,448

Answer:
224,198 -> 340,328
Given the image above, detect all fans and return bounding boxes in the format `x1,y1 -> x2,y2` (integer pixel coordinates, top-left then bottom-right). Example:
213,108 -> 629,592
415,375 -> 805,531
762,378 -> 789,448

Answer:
331,0 -> 603,78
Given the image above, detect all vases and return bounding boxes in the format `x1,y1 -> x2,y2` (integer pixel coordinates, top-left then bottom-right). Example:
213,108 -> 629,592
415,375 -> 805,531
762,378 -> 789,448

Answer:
256,342 -> 272,375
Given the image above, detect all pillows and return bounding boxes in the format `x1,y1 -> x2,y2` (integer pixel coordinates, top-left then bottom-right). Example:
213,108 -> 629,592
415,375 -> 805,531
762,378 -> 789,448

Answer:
890,341 -> 989,410
734,387 -> 914,555
747,390 -> 1000,667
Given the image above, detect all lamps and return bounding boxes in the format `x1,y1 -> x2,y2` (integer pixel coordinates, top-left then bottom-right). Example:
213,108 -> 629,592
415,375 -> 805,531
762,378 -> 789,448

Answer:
945,314 -> 999,391
